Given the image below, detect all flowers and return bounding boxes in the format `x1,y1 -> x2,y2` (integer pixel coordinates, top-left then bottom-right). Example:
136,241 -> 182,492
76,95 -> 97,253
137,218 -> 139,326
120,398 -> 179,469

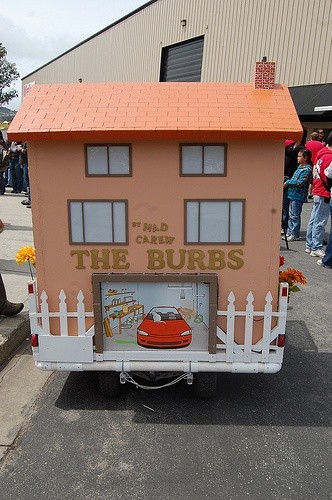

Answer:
16,246 -> 37,279
278,255 -> 307,295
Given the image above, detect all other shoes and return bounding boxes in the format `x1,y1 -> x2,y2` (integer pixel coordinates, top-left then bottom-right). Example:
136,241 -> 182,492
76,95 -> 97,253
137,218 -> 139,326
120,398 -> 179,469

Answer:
305,248 -> 311,253
310,248 -> 325,257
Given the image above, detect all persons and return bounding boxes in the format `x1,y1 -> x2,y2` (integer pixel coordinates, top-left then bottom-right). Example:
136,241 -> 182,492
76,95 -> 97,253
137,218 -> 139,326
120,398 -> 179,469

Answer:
281,139 -> 313,241
0,273 -> 24,318
305,129 -> 332,269
0,130 -> 30,195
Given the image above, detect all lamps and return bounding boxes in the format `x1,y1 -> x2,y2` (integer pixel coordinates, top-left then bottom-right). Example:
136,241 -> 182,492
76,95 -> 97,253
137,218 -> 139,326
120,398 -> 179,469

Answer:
180,19 -> 186,27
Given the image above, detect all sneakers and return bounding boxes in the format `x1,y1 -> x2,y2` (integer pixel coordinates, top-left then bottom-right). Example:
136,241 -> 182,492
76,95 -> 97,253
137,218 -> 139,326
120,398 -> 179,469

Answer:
281,233 -> 290,240
287,236 -> 299,241
0,300 -> 24,317
317,259 -> 331,268
